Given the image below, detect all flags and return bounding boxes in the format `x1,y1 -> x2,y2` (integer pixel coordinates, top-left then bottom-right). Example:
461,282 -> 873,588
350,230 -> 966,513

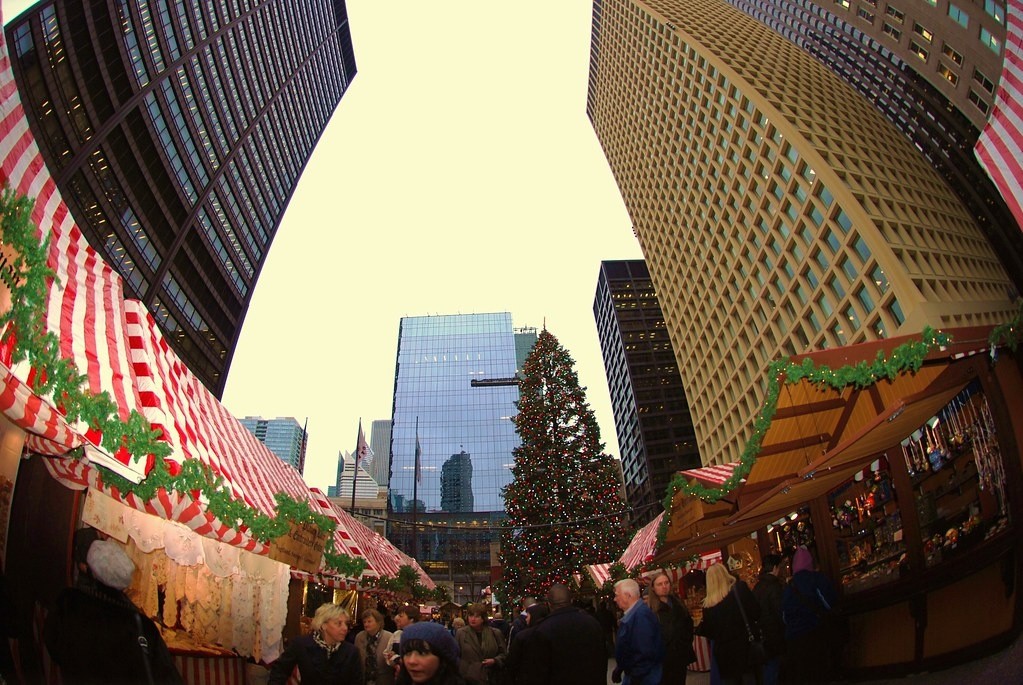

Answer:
417,435 -> 421,484
353,425 -> 367,483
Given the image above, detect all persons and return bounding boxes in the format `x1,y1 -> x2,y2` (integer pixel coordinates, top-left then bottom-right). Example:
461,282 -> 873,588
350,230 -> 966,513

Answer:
855,559 -> 873,574
782,550 -> 835,685
267,585 -> 617,685
752,554 -> 789,685
613,572 -> 694,685
43,528 -> 185,685
694,563 -> 765,685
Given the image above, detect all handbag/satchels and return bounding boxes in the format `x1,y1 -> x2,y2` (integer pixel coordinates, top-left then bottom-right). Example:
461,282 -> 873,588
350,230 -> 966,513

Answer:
733,583 -> 787,667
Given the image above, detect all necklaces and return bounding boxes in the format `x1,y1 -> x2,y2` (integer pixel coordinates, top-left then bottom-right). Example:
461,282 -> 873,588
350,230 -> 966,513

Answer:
902,388 -> 1006,515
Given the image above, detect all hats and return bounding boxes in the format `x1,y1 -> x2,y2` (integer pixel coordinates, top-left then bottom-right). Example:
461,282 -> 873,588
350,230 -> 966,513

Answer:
495,613 -> 503,620
792,544 -> 814,573
86,539 -> 135,590
399,622 -> 461,662
